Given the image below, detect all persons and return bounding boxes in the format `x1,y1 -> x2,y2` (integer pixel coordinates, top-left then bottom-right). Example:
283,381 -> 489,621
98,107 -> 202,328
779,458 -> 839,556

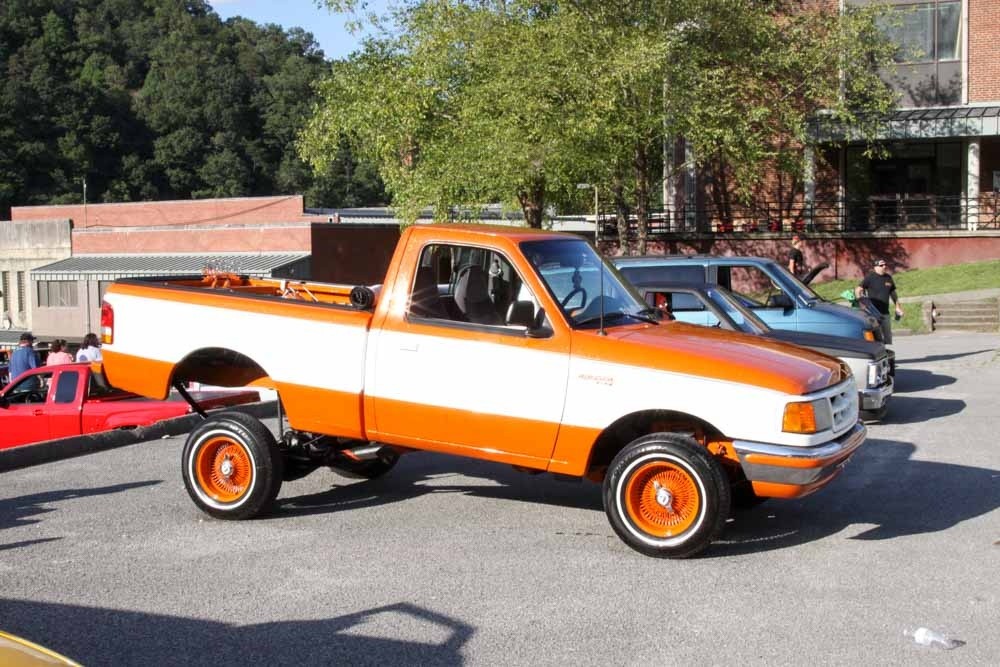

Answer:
46,340 -> 73,366
8,334 -> 44,402
788,236 -> 803,279
76,334 -> 101,362
855,258 -> 903,344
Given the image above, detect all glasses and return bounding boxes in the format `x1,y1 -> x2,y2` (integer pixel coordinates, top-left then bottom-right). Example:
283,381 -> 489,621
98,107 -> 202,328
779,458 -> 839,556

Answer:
874,264 -> 888,267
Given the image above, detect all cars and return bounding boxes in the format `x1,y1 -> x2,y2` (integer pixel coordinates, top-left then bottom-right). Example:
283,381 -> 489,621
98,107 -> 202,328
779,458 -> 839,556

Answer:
370,255 -> 898,425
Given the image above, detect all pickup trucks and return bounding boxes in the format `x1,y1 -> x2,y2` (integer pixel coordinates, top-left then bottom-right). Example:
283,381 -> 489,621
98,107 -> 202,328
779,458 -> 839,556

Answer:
86,220 -> 871,560
1,361 -> 262,452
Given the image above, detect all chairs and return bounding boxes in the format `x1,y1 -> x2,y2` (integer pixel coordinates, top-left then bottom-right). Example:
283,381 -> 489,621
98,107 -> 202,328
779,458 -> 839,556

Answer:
454,263 -> 504,325
415,265 -> 450,320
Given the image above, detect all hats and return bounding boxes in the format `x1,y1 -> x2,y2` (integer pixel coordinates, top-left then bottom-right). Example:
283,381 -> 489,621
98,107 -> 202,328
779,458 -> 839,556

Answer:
20,333 -> 36,340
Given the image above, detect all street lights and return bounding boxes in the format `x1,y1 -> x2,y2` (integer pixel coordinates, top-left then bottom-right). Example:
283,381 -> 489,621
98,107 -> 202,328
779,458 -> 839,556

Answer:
576,182 -> 600,250
